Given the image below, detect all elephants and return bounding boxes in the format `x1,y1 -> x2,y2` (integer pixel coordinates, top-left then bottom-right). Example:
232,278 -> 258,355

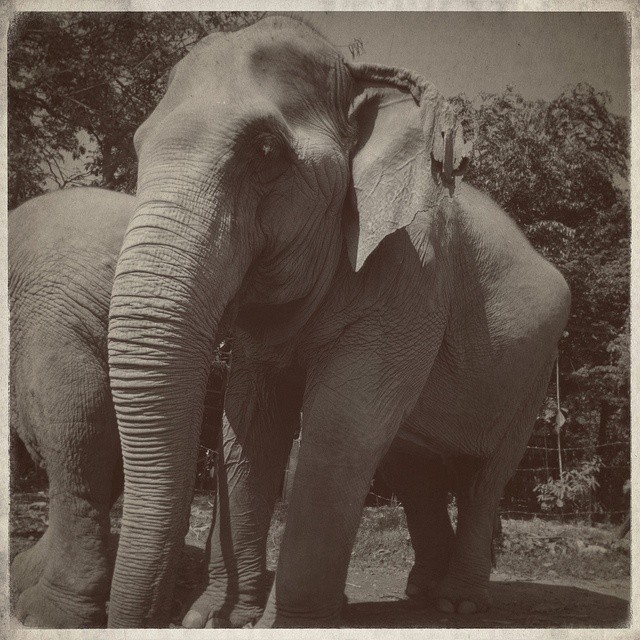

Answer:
8,12 -> 574,629
